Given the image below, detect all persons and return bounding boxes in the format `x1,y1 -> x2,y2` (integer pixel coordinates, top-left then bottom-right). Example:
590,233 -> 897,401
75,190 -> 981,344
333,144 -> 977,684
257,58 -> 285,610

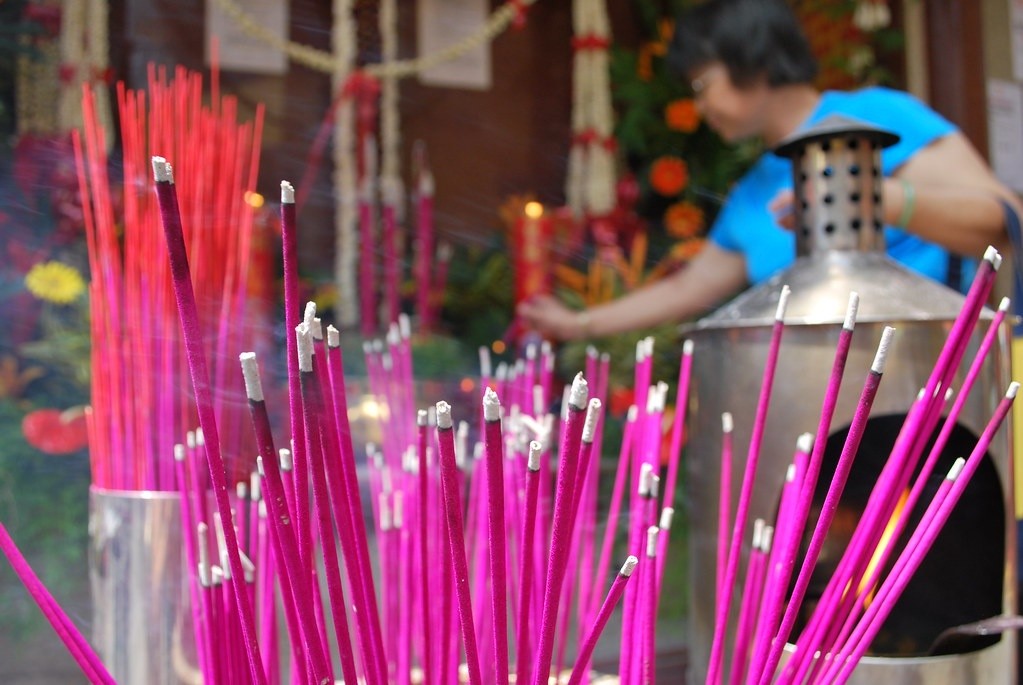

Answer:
513,0 -> 1023,342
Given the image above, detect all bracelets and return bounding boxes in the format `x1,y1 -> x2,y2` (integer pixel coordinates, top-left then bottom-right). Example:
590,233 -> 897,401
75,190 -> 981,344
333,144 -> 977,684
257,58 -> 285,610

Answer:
897,175 -> 915,229
